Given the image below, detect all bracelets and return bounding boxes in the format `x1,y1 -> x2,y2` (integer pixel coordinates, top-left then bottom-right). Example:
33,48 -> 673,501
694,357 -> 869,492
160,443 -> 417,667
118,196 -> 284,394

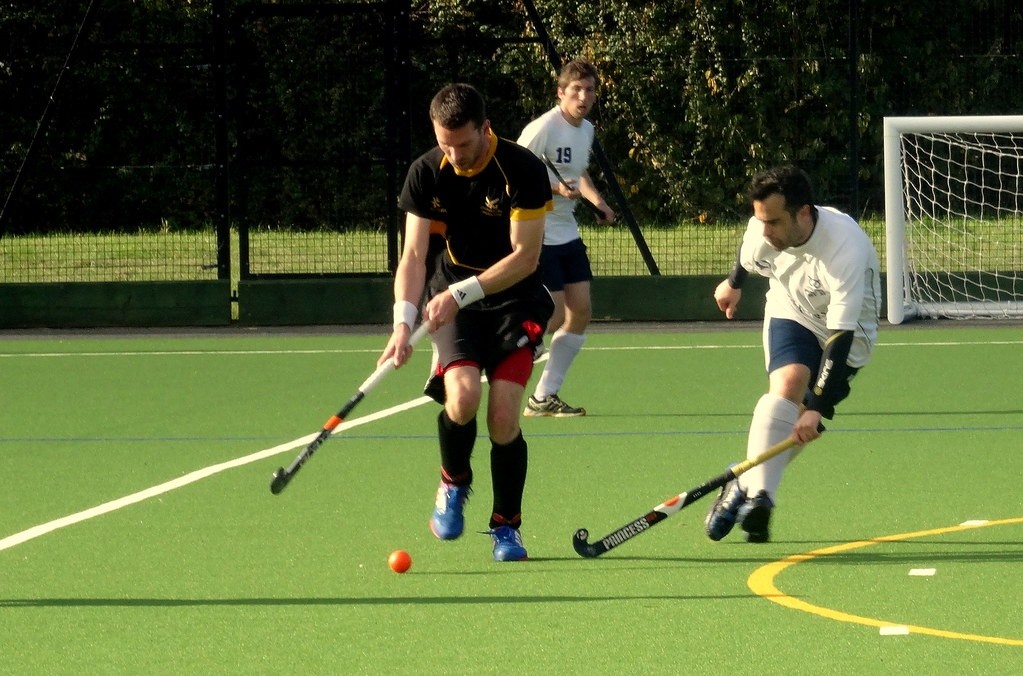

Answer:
393,301 -> 418,334
448,276 -> 485,309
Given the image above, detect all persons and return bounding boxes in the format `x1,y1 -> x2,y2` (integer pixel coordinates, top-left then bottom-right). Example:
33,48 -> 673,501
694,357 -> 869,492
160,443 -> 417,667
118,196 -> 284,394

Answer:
375,84 -> 557,561
705,163 -> 881,541
516,56 -> 615,417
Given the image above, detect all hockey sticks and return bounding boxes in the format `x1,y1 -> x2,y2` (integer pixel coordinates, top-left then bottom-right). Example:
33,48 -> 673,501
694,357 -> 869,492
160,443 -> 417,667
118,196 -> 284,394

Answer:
269,316 -> 431,497
570,421 -> 828,560
541,150 -> 620,229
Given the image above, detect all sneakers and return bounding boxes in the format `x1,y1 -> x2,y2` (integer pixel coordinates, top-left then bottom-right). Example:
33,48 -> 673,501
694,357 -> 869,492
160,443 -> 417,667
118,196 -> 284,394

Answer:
703,463 -> 747,542
429,478 -> 472,541
475,526 -> 529,562
735,489 -> 775,543
522,394 -> 586,418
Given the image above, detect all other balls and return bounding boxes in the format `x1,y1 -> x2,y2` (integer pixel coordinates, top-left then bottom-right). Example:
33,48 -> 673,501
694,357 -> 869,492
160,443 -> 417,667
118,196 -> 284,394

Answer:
388,549 -> 414,574
